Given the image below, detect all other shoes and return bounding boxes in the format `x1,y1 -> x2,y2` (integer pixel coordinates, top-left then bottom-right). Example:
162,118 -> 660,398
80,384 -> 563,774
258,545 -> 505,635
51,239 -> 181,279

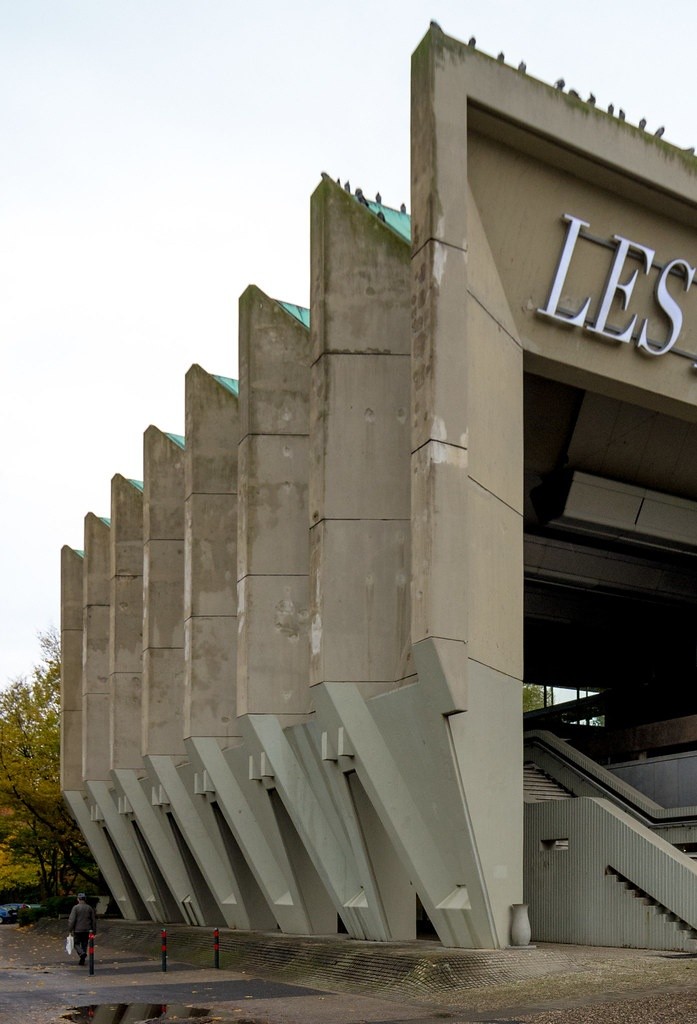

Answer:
78,962 -> 85,966
80,952 -> 88,959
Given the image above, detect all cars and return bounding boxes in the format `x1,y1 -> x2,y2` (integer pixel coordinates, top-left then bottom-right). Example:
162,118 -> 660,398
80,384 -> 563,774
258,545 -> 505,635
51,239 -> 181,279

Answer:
0,903 -> 46,924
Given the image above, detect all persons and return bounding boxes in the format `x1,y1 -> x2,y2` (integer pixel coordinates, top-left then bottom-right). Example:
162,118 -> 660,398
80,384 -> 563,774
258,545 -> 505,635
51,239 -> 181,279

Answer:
68,893 -> 97,964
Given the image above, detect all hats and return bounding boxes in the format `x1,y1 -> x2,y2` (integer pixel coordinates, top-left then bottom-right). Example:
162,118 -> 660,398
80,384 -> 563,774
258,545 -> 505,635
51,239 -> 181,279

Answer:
76,893 -> 86,898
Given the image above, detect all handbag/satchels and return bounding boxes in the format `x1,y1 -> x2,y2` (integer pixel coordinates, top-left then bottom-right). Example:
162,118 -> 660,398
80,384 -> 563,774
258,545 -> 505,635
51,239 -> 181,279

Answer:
65,934 -> 74,955
